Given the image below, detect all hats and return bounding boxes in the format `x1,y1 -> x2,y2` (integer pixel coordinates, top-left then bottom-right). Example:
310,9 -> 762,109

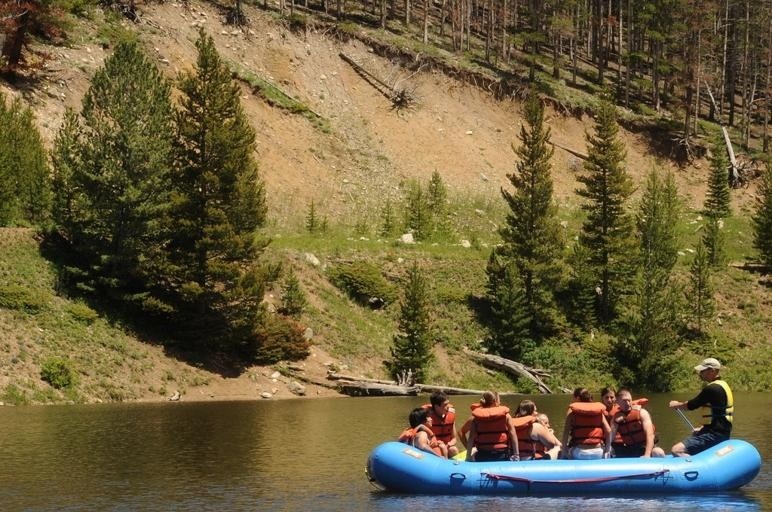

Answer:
695,358 -> 720,371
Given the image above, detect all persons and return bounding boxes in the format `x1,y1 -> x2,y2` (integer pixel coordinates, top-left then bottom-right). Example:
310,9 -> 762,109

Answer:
401,385 -> 665,461
669,357 -> 734,458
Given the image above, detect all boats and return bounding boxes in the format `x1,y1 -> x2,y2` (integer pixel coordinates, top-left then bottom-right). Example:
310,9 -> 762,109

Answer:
364,438 -> 763,496
